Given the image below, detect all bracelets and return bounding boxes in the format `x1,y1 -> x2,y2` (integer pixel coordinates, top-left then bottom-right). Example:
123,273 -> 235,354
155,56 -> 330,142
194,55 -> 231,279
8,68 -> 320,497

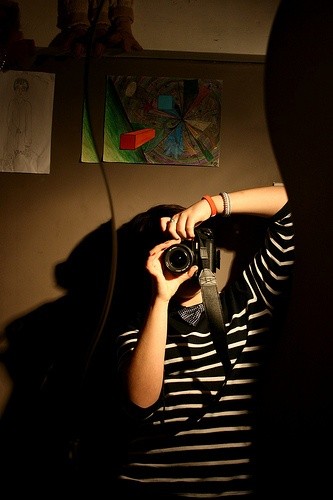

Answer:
201,195 -> 218,217
219,192 -> 230,217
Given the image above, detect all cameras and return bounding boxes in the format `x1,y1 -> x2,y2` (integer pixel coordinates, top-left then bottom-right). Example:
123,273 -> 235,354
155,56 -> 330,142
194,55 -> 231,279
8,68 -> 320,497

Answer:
163,230 -> 200,275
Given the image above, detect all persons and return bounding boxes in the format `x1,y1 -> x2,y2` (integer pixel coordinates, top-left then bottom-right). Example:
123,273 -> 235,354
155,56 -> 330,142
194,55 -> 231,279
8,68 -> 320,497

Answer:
113,186 -> 295,500
